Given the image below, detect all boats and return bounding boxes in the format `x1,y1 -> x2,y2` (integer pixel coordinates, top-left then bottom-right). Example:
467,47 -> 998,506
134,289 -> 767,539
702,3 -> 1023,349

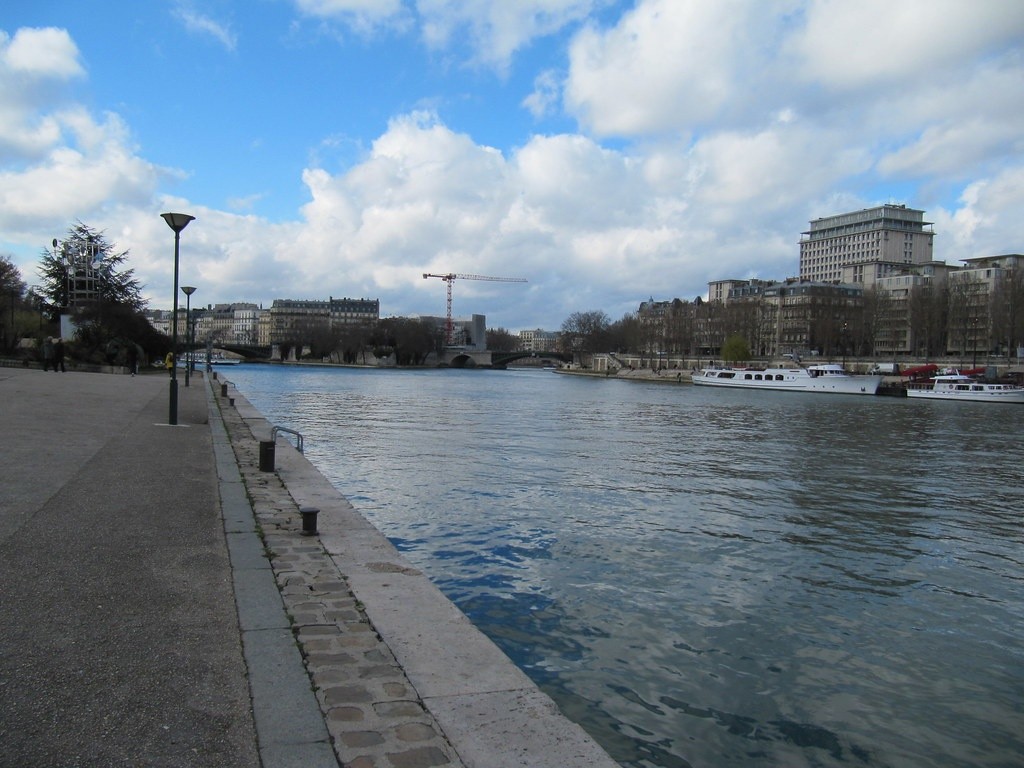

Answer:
689,364 -> 886,396
902,362 -> 1024,405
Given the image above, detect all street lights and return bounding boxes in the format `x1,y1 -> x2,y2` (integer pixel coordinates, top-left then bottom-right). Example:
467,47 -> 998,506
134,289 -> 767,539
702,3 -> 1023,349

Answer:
189,321 -> 199,376
160,212 -> 196,425
972,318 -> 978,368
180,286 -> 197,386
843,322 -> 847,369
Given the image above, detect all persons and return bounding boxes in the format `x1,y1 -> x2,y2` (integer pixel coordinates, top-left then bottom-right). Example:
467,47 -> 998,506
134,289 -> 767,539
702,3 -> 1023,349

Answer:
44,336 -> 57,371
128,345 -> 140,377
165,349 -> 173,377
54,338 -> 67,373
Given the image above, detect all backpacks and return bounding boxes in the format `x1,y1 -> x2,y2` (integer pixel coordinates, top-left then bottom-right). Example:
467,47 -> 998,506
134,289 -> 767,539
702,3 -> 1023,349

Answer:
169,354 -> 173,362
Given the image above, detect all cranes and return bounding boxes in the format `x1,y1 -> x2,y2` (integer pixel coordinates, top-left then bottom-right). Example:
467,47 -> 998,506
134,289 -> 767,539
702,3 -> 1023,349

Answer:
423,272 -> 526,345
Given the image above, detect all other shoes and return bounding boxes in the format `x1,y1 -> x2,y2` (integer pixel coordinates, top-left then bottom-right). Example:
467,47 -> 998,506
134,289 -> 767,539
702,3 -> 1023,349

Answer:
132,372 -> 135,378
